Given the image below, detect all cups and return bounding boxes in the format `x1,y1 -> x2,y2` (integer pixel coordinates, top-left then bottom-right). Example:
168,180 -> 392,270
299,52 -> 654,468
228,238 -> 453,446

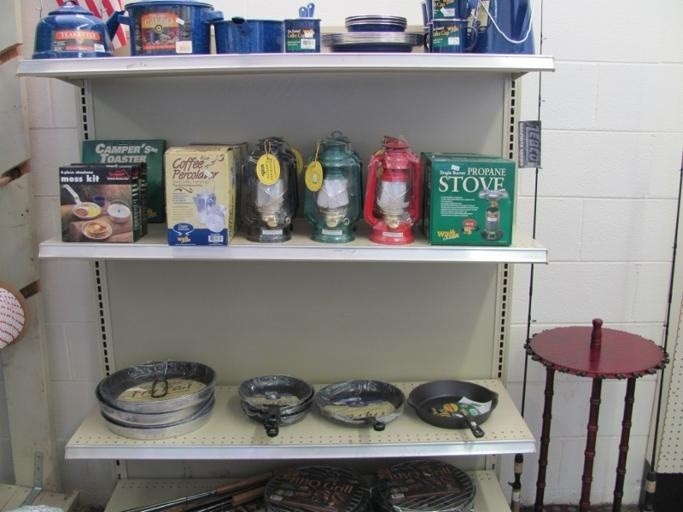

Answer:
281,16 -> 322,53
430,18 -> 478,55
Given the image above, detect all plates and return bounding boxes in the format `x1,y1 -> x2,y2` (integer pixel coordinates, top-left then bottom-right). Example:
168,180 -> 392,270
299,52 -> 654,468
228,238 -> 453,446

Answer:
322,14 -> 424,52
80,220 -> 113,241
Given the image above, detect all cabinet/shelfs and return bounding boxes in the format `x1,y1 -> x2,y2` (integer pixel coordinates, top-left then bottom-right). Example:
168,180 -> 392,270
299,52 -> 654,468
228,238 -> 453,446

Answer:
524,326 -> 668,512
14,52 -> 555,512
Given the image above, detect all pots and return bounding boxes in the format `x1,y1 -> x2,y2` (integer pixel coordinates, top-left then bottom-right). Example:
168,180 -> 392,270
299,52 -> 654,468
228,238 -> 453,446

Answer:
407,379 -> 500,438
238,373 -> 317,438
314,379 -> 406,432
211,16 -> 285,55
61,182 -> 101,220
117,0 -> 224,56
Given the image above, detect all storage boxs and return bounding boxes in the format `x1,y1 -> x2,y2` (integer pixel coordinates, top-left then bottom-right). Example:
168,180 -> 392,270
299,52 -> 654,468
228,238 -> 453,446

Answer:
59,139 -> 251,244
420,150 -> 516,246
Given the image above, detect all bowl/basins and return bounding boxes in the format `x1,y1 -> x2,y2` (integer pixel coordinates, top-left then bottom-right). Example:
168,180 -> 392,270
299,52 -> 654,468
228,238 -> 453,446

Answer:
95,358 -> 217,440
106,203 -> 131,223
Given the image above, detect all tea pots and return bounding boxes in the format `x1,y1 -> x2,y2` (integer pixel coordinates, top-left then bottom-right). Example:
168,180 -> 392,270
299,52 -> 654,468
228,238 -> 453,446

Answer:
31,0 -> 125,59
